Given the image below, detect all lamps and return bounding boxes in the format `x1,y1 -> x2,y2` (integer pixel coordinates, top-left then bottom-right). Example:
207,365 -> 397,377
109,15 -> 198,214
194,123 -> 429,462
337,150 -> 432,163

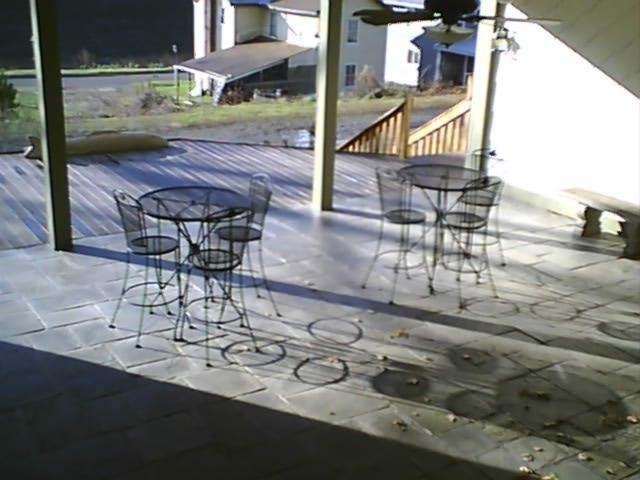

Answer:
423,22 -> 475,48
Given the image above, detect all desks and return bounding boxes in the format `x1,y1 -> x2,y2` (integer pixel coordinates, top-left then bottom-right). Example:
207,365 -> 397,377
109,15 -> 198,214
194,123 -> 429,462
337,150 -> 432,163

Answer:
136,186 -> 253,341
397,164 -> 488,294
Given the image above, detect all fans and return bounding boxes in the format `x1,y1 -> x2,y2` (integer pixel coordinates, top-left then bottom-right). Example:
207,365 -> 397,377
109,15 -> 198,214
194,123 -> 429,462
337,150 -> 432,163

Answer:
352,0 -> 562,26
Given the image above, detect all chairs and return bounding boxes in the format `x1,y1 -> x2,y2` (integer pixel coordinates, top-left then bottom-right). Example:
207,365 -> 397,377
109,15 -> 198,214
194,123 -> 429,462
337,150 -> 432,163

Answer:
430,176 -> 502,308
111,188 -> 180,347
213,173 -> 281,316
454,148 -> 503,245
362,164 -> 431,305
173,207 -> 259,367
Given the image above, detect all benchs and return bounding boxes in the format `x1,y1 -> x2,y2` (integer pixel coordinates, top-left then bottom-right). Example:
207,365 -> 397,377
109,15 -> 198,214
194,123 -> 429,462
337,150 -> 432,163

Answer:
560,187 -> 640,257
